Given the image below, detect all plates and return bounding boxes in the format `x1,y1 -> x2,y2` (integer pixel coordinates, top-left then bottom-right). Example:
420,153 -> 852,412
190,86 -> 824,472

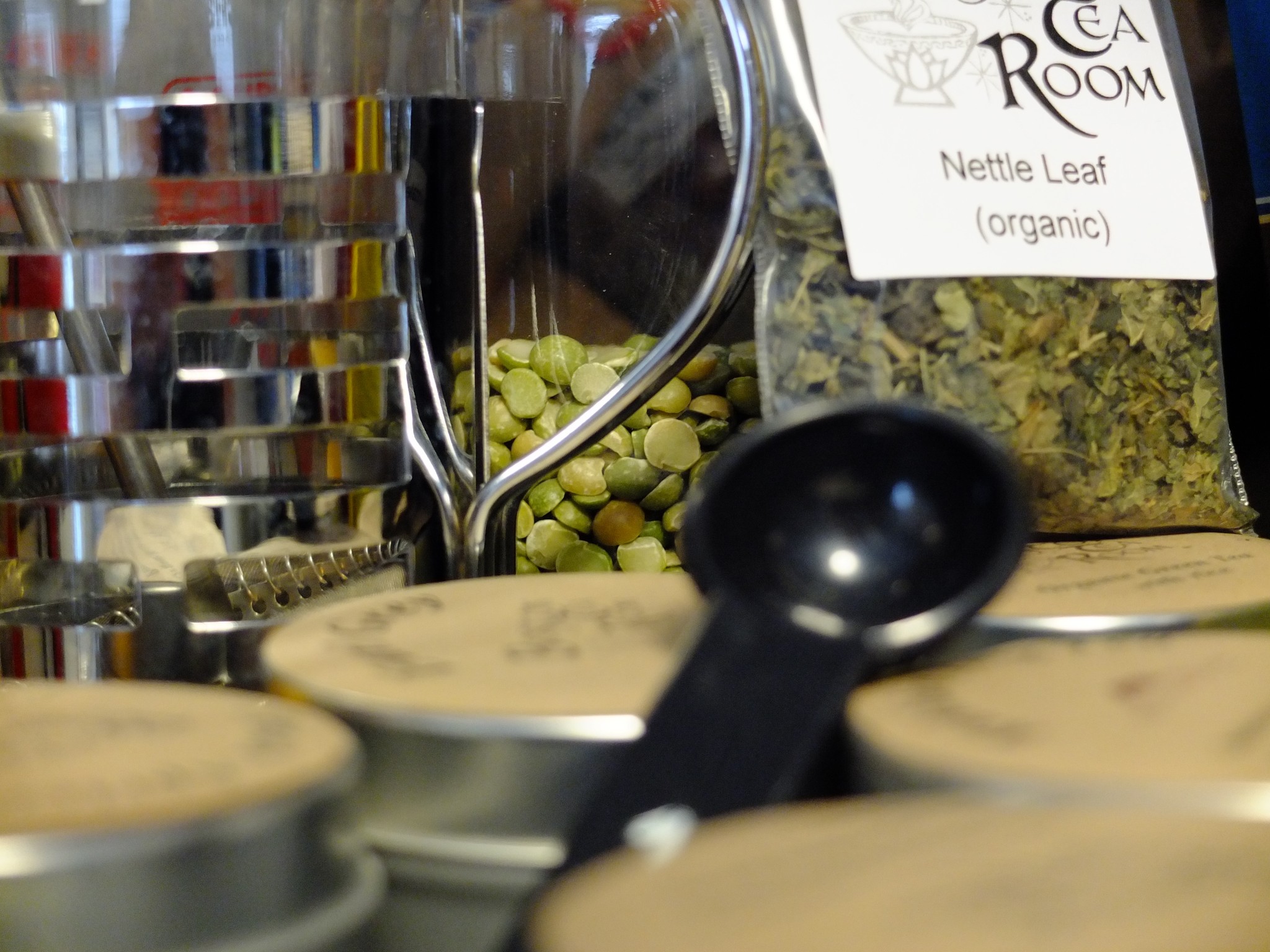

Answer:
527,785 -> 1269,952
260,569 -> 709,743
846,622 -> 1270,783
1,667 -> 362,830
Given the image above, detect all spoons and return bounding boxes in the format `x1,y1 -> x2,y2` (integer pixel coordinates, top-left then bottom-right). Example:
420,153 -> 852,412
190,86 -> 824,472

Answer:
495,394 -> 1034,952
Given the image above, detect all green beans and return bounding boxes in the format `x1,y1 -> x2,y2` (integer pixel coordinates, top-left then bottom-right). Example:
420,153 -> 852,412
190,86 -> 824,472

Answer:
442,323 -> 762,574
